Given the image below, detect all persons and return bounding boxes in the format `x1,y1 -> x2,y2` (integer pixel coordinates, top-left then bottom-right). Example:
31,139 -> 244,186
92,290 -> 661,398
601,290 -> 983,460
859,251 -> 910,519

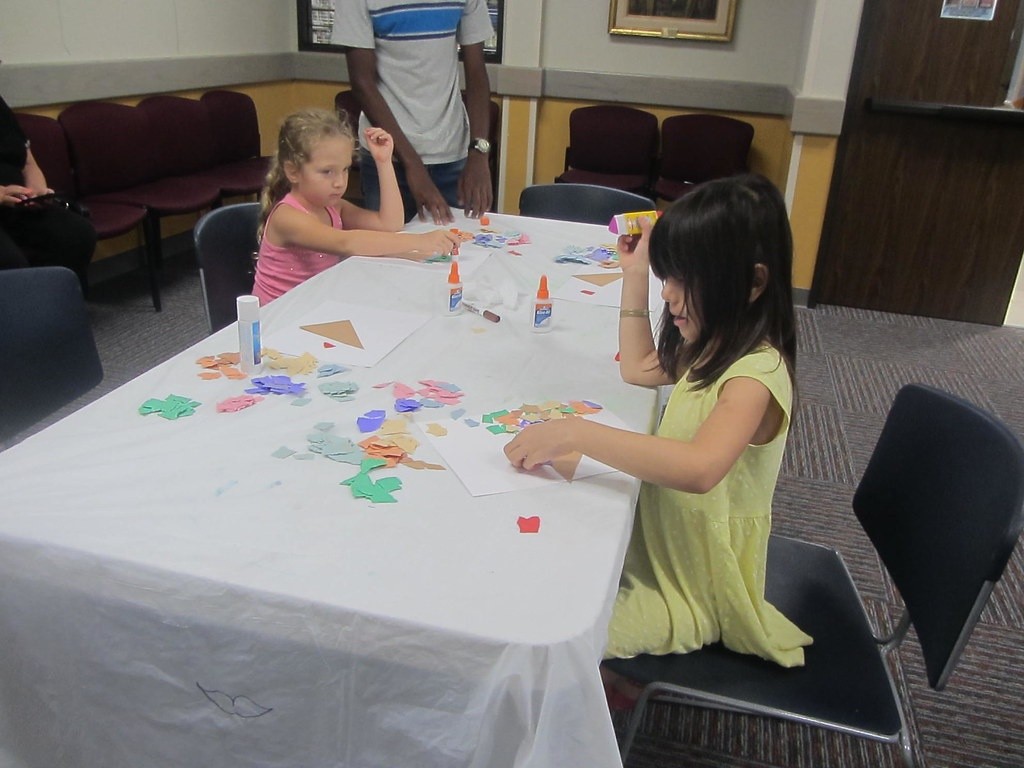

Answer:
328,0 -> 495,226
0,96 -> 96,285
248,108 -> 462,308
504,172 -> 815,719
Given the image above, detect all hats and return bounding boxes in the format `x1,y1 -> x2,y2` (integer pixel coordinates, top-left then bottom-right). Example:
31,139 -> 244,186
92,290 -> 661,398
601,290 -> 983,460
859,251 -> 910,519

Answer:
0,194 -> 68,251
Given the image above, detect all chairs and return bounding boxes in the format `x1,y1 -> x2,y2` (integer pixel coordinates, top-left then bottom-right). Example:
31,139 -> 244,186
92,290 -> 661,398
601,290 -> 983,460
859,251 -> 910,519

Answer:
649,113 -> 754,204
193,202 -> 262,336
10,89 -> 275,312
0,267 -> 104,454
554,104 -> 659,202
519,184 -> 657,226
614,381 -> 1024,768
334,89 -> 497,207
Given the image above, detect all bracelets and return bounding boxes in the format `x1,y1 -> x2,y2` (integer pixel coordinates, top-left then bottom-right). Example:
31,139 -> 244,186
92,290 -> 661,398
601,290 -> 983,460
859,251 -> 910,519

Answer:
620,309 -> 649,318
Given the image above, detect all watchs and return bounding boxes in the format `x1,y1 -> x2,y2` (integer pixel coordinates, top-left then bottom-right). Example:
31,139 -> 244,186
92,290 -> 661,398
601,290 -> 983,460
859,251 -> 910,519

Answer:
468,137 -> 492,154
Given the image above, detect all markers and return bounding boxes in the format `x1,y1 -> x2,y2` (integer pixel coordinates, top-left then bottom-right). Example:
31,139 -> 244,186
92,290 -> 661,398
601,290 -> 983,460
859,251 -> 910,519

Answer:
462,298 -> 501,323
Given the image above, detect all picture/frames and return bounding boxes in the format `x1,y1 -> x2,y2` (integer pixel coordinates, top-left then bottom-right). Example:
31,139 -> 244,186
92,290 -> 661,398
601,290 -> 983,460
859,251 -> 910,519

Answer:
607,0 -> 737,43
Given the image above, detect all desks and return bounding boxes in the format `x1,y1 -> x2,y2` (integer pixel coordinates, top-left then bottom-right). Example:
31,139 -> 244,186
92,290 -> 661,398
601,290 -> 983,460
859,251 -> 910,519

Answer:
1,203 -> 672,768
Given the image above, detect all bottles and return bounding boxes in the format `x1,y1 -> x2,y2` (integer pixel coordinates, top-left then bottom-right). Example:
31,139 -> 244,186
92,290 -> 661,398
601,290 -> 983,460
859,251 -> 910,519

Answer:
236,295 -> 263,376
443,261 -> 462,315
531,275 -> 553,332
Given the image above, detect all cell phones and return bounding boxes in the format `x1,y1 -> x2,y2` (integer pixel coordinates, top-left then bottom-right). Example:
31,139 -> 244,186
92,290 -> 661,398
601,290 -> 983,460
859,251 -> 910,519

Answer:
15,192 -> 59,207
